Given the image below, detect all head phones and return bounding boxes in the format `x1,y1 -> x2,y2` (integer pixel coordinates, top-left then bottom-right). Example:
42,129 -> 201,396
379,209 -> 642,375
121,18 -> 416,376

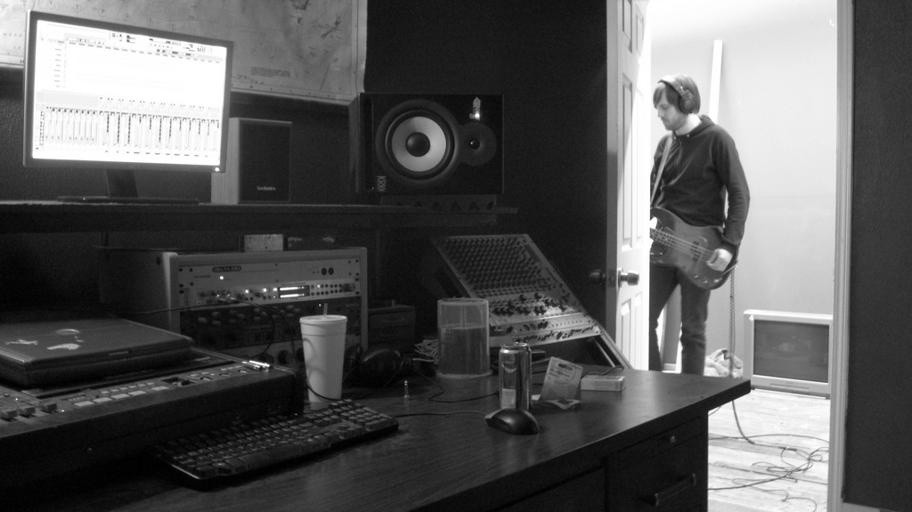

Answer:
657,76 -> 699,112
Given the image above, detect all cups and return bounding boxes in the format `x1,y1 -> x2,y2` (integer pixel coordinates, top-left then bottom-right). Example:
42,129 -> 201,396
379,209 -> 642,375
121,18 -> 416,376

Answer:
299,314 -> 348,409
436,297 -> 490,375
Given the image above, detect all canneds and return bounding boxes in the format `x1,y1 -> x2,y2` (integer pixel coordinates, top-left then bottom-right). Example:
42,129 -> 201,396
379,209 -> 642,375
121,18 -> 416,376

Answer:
498,342 -> 533,416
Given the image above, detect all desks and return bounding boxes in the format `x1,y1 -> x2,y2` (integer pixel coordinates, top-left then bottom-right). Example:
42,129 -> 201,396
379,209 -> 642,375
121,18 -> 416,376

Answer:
1,201 -> 751,512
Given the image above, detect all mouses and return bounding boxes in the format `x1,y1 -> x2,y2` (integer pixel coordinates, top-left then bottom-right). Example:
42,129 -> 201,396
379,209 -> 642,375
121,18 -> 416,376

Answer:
485,409 -> 540,434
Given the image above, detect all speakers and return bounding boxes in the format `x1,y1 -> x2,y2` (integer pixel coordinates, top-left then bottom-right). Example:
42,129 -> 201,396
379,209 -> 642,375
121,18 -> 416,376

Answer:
345,91 -> 503,203
210,118 -> 292,202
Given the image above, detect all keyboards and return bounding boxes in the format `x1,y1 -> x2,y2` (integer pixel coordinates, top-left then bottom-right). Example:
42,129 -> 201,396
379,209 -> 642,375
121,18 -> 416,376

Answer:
148,398 -> 398,489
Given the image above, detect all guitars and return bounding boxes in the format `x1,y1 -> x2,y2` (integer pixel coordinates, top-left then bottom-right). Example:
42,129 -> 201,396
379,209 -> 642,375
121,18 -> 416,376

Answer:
649,207 -> 734,290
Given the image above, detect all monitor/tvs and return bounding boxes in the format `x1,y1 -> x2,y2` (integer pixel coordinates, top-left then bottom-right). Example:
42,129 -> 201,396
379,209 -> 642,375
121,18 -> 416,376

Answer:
742,310 -> 832,402
23,9 -> 234,204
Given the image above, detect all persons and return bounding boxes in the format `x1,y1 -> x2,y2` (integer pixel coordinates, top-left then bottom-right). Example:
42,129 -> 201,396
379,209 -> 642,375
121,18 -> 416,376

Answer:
648,72 -> 751,375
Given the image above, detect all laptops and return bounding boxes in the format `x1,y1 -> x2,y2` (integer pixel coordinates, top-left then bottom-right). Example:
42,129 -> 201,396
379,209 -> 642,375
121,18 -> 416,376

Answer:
0,317 -> 196,390
